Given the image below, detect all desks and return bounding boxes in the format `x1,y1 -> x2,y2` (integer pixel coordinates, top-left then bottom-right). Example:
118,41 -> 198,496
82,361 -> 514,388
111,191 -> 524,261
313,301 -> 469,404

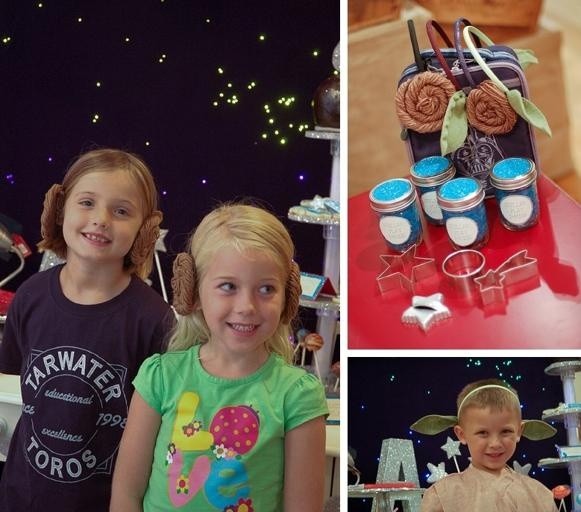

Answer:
347,164 -> 579,350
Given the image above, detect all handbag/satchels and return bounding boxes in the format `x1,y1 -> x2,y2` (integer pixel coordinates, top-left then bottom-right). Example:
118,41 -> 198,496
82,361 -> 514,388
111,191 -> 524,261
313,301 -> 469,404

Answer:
394,18 -> 552,200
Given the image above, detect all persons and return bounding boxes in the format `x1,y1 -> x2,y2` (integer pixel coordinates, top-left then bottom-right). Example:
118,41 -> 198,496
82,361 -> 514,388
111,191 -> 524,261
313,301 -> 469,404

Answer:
420,380 -> 558,511
0,148 -> 178,512
108,204 -> 329,511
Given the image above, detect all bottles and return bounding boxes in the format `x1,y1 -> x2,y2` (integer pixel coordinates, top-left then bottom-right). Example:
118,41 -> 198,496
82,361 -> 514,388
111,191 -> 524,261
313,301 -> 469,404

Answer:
369,178 -> 423,253
436,176 -> 490,251
408,156 -> 456,226
489,156 -> 540,232
